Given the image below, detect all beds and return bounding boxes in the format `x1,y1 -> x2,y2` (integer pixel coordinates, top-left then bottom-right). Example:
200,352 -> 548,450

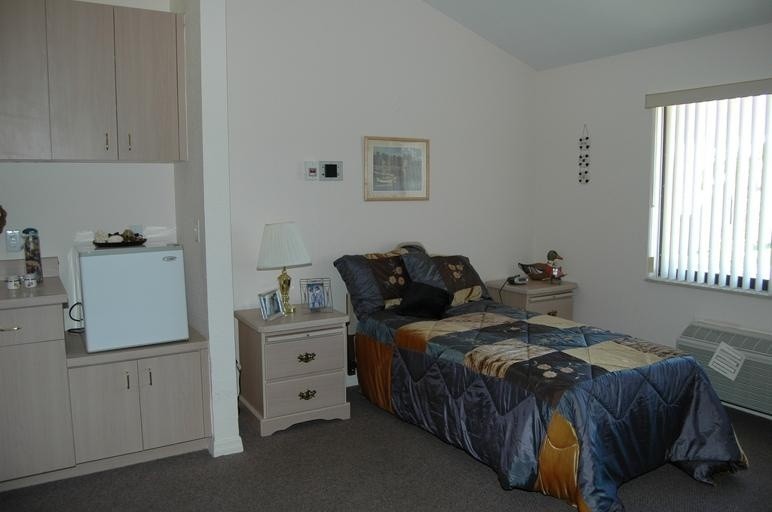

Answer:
346,293 -> 749,512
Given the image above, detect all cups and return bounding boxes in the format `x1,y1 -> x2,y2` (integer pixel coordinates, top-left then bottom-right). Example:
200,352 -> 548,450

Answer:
8,280 -> 21,290
24,278 -> 37,289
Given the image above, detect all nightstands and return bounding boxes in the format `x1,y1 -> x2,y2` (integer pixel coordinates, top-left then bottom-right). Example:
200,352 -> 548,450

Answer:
233,303 -> 351,437
486,277 -> 577,320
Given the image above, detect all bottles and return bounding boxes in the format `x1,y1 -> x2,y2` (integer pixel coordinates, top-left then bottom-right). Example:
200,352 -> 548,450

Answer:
24,234 -> 44,284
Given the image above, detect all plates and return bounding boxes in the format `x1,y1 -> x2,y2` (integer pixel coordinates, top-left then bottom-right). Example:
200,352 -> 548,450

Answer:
93,239 -> 147,247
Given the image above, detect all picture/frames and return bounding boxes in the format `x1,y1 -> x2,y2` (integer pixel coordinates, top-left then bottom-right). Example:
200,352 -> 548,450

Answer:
364,136 -> 430,202
300,278 -> 333,314
259,288 -> 285,322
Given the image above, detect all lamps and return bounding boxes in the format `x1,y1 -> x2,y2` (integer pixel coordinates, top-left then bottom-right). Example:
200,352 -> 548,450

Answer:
256,221 -> 311,313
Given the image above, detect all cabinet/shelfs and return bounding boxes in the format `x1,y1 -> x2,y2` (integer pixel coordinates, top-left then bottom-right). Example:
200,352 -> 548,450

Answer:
0,295 -> 76,492
0,0 -> 53,162
46,0 -> 188,164
67,341 -> 212,477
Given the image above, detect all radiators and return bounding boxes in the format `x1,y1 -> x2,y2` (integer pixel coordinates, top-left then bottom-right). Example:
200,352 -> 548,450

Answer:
678,320 -> 772,421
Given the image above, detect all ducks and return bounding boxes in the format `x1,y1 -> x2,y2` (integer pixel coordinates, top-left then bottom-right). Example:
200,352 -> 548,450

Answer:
518,250 -> 564,281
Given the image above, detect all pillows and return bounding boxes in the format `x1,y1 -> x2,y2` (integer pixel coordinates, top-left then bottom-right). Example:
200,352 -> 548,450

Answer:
334,241 -> 447,321
432,255 -> 492,306
397,282 -> 454,321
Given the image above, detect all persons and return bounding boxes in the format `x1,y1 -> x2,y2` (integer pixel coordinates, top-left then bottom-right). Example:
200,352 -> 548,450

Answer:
309,287 -> 315,309
315,286 -> 323,308
